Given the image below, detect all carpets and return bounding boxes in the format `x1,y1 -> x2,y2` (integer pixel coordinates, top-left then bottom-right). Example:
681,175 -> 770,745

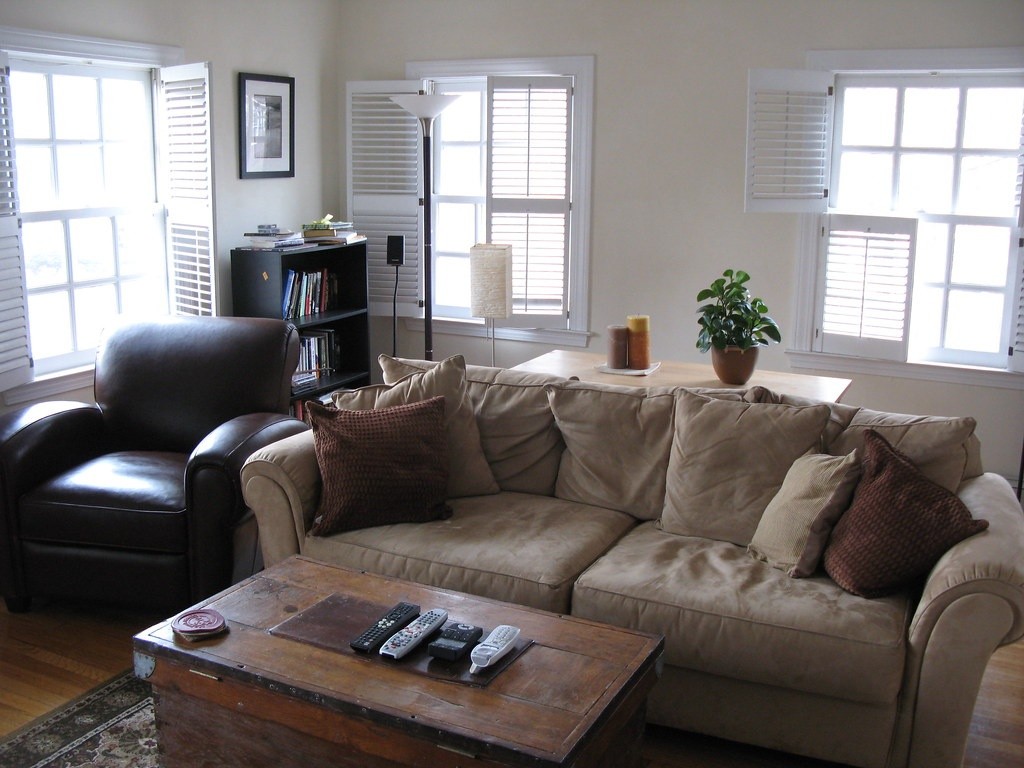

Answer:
0,670 -> 160,768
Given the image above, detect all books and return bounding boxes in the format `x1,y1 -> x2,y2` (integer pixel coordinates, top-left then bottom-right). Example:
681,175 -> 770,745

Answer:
289,399 -> 303,421
244,213 -> 366,252
283,268 -> 328,321
291,329 -> 341,395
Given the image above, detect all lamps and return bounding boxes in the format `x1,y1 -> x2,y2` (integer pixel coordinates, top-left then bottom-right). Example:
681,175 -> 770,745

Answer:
469,243 -> 513,369
388,91 -> 461,363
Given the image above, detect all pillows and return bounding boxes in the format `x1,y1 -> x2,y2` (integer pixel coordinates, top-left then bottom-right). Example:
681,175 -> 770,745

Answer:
823,430 -> 990,597
303,396 -> 456,535
655,387 -> 832,550
330,354 -> 501,499
747,447 -> 863,578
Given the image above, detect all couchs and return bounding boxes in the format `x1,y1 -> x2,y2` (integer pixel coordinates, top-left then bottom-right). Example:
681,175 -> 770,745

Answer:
0,314 -> 311,616
242,360 -> 1024,768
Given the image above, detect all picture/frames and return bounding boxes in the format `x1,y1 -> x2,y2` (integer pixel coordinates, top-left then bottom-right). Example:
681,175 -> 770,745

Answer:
238,71 -> 296,182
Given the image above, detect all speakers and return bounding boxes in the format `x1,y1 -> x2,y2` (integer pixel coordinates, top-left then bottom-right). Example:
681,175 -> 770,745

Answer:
386,235 -> 405,266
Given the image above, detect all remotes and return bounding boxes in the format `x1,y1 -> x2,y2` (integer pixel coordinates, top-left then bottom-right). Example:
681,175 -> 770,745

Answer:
349,603 -> 421,655
470,624 -> 521,675
379,608 -> 448,659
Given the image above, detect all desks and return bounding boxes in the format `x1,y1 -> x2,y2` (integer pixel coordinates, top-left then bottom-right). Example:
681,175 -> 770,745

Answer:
133,552 -> 666,768
507,350 -> 853,405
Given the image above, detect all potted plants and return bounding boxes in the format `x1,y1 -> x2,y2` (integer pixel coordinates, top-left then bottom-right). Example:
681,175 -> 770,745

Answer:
694,269 -> 780,384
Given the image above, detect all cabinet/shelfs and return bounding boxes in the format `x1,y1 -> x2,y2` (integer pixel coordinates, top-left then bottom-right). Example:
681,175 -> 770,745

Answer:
230,235 -> 372,419
